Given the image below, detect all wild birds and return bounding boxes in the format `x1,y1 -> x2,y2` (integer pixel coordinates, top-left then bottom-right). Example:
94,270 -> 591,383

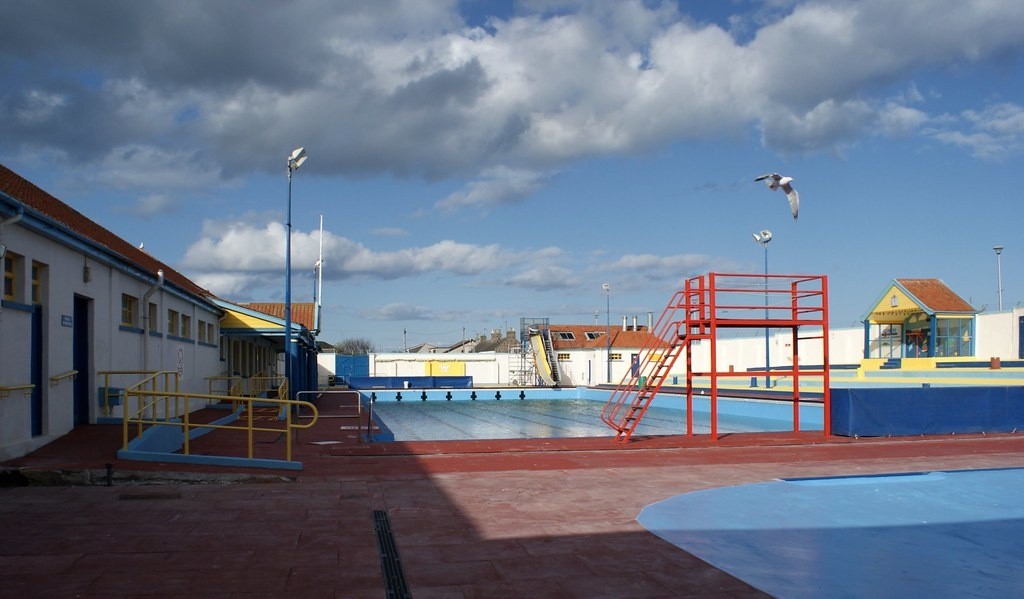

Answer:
754,172 -> 801,224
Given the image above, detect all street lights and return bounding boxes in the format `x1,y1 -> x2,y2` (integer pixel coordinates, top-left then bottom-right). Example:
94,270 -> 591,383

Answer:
752,230 -> 773,387
602,283 -> 611,383
993,246 -> 1004,311
286,148 -> 308,403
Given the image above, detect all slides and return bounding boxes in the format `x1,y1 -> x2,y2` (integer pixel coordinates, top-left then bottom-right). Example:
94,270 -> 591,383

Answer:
528,332 -> 557,385
